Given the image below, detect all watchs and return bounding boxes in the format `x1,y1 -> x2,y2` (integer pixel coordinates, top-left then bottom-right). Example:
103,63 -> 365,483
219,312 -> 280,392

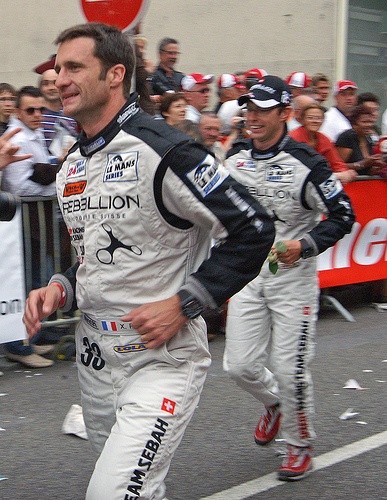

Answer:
176,289 -> 202,319
299,238 -> 312,259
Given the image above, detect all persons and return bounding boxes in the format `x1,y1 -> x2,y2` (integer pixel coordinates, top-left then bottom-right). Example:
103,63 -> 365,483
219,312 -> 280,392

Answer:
19,22 -> 276,500
0,37 -> 387,367
222,75 -> 355,481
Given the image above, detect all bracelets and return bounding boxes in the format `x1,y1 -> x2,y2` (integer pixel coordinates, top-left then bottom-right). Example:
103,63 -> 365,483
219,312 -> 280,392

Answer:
48,281 -> 66,308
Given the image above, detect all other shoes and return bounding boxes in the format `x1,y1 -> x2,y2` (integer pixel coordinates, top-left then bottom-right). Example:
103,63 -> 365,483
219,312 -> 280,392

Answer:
279,440 -> 312,480
254,403 -> 282,446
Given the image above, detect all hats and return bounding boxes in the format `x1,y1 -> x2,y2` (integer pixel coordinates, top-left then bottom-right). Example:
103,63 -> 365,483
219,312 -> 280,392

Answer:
217,73 -> 242,87
285,72 -> 312,88
181,73 -> 215,90
336,79 -> 357,92
236,75 -> 293,111
244,67 -> 267,85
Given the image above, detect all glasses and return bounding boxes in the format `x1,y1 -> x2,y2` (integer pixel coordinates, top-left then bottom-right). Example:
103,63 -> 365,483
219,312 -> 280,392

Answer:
188,88 -> 209,93
160,49 -> 182,55
18,107 -> 47,114
